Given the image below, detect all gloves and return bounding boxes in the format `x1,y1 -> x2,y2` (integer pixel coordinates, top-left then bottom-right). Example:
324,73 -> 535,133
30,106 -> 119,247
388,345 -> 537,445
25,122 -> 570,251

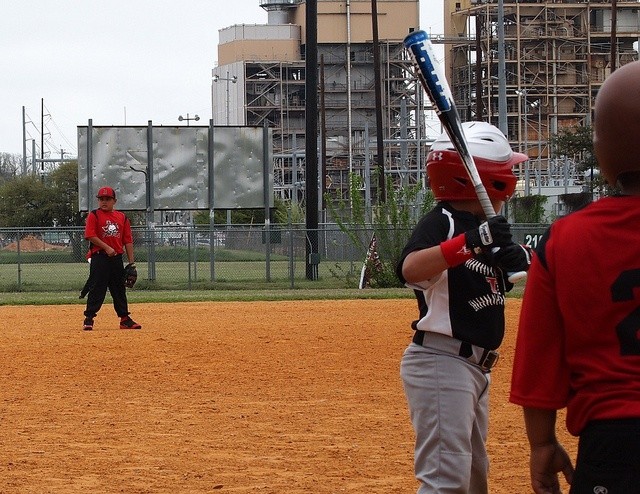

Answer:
465,215 -> 513,258
491,243 -> 534,272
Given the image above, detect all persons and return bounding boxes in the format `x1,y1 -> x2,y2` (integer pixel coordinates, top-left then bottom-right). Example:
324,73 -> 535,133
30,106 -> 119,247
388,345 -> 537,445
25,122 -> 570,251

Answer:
509,61 -> 640,494
395,121 -> 532,494
79,187 -> 142,330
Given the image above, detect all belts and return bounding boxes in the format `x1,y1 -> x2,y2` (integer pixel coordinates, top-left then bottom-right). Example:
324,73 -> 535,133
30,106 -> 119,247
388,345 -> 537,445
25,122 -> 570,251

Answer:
413,330 -> 499,369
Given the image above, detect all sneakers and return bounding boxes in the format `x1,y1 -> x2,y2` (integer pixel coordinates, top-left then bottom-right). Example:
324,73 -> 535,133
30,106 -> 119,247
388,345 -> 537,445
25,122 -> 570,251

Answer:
82,316 -> 94,330
120,316 -> 142,329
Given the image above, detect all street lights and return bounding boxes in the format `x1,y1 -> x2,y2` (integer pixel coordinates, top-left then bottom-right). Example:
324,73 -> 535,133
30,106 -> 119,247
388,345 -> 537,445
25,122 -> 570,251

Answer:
213,71 -> 238,230
178,112 -> 200,224
530,99 -> 542,195
515,88 -> 528,197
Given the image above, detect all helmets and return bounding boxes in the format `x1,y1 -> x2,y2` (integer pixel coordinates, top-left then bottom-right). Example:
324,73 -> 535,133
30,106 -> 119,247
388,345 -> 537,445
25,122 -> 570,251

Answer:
425,121 -> 528,202
595,60 -> 640,188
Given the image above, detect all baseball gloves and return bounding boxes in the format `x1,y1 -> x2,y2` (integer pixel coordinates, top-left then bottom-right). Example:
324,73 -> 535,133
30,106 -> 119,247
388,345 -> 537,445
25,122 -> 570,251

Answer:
122,262 -> 138,288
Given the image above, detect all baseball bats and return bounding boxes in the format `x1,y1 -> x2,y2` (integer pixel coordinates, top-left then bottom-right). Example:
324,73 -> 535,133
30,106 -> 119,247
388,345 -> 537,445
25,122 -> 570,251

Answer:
403,28 -> 528,283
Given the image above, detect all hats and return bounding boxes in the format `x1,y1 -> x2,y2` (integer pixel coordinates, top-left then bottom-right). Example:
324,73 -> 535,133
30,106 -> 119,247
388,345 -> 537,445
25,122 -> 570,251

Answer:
96,186 -> 115,198
